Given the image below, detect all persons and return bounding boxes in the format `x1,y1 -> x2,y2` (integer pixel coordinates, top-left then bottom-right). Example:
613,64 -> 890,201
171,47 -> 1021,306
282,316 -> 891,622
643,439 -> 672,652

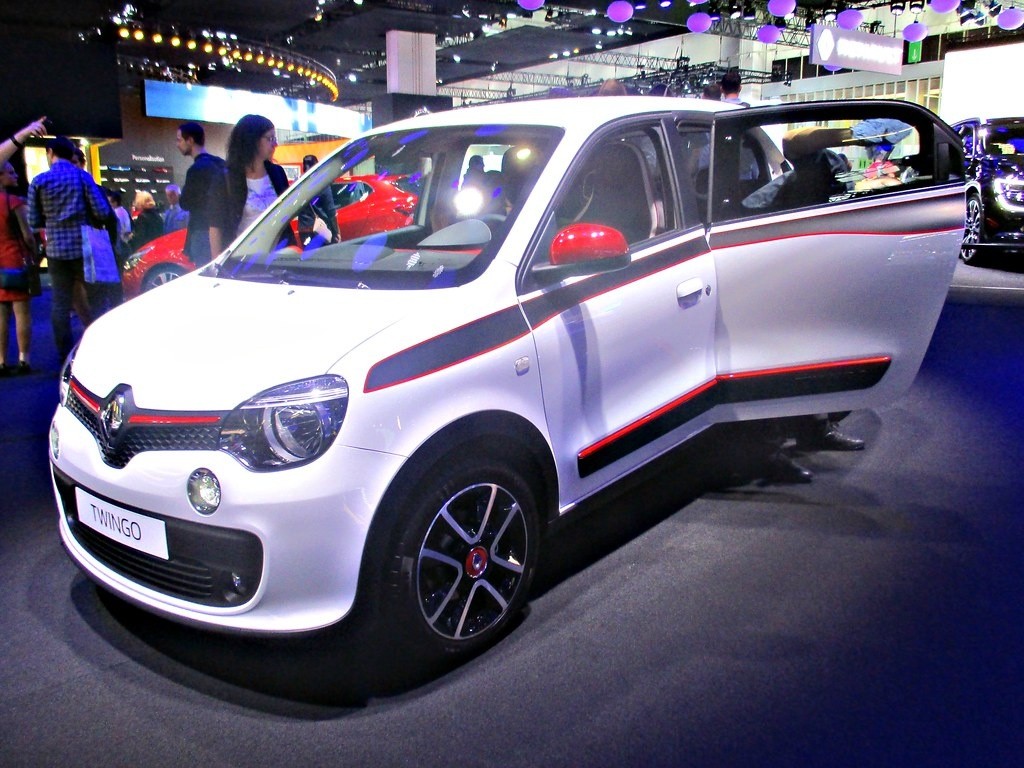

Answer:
0,116 -> 190,379
177,115 -> 341,270
720,119 -> 914,488
461,153 -> 485,189
599,72 -> 760,179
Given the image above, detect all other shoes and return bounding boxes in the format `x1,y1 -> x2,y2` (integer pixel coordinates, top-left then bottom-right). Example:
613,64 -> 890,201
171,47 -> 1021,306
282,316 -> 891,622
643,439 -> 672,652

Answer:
0,360 -> 31,374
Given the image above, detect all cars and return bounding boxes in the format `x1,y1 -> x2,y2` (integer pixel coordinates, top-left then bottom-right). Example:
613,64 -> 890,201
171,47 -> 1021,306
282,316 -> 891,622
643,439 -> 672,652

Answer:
950,117 -> 1024,268
49,96 -> 966,677
121,173 -> 420,303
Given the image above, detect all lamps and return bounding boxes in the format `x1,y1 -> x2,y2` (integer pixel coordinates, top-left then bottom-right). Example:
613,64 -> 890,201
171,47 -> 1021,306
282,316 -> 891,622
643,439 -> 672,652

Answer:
890,0 -> 906,16
910,0 -> 925,14
806,19 -> 817,31
462,1 -> 517,34
956,0 -> 1002,27
660,0 -> 671,7
117,20 -> 339,102
635,0 -> 646,9
545,7 -> 634,59
775,17 -> 785,30
823,3 -> 837,21
707,0 -> 755,20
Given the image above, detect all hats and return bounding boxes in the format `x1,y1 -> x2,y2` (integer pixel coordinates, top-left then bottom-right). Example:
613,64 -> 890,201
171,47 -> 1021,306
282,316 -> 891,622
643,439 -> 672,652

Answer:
303,156 -> 318,167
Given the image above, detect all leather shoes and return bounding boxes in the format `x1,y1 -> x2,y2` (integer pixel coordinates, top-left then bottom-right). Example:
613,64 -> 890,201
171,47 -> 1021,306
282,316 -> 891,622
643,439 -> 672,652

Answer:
763,454 -> 815,483
799,424 -> 865,452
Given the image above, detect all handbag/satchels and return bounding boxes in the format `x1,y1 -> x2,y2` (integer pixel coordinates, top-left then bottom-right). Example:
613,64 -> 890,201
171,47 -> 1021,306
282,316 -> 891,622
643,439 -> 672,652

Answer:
190,230 -> 229,262
81,223 -> 122,286
24,264 -> 42,295
313,217 -> 333,245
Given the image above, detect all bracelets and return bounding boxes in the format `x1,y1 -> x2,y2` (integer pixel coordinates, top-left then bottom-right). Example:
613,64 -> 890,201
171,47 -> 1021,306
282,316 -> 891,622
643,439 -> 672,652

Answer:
9,136 -> 25,150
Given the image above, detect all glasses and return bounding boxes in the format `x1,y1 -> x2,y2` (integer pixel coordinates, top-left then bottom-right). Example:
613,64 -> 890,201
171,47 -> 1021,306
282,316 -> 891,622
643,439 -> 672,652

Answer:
263,136 -> 276,144
